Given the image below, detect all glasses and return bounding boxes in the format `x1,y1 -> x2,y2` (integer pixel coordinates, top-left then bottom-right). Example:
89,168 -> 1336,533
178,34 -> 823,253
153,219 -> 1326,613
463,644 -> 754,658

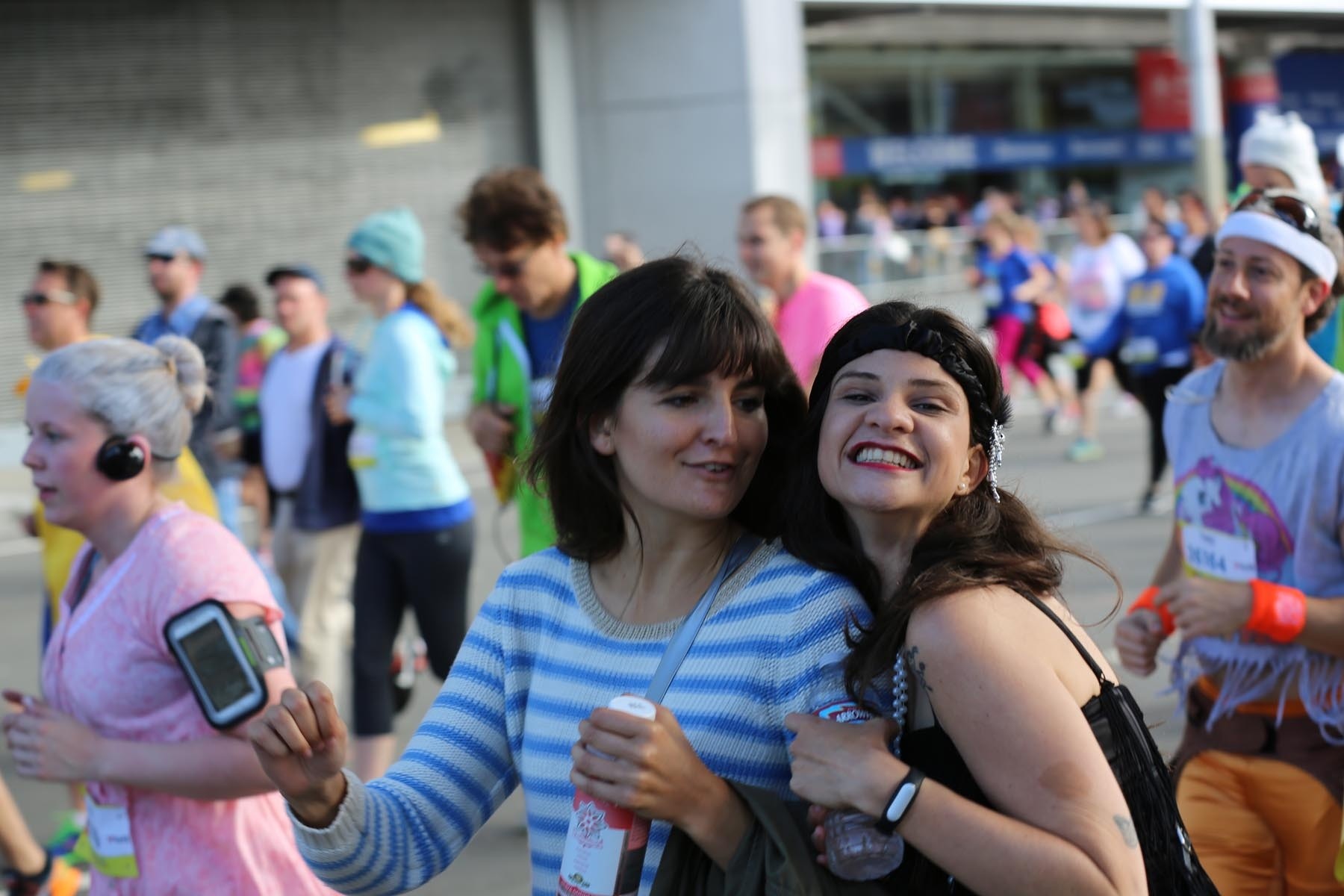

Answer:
1233,188 -> 1324,242
22,290 -> 78,308
144,251 -> 176,262
343,256 -> 371,275
471,240 -> 544,280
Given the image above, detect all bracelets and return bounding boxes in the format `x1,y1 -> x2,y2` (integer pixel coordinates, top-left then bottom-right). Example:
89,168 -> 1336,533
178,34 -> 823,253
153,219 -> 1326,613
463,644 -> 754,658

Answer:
876,766 -> 927,838
1128,585 -> 1177,636
1243,578 -> 1307,646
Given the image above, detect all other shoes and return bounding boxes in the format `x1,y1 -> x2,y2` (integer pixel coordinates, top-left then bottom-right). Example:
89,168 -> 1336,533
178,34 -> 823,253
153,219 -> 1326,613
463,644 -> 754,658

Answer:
388,640 -> 415,714
1067,442 -> 1104,461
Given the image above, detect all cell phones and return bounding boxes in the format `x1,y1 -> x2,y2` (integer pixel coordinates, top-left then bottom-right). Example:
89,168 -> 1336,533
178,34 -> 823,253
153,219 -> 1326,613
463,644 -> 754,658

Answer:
162,596 -> 270,733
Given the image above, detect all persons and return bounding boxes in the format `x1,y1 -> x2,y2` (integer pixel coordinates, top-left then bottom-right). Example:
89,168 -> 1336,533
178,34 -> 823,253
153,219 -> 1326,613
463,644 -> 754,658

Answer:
244,257 -> 881,896
785,300 -> 1219,896
1115,185 -> 1344,896
819,108 -> 1344,523
459,167 -> 876,556
0,212 -> 475,896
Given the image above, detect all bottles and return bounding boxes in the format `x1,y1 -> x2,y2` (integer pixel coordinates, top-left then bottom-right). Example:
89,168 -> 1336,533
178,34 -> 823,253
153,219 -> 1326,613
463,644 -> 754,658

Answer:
557,694 -> 656,896
806,651 -> 903,881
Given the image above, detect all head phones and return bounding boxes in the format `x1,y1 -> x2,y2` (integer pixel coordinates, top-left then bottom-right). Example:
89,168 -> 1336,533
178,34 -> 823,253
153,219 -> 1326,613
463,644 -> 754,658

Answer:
96,433 -> 183,481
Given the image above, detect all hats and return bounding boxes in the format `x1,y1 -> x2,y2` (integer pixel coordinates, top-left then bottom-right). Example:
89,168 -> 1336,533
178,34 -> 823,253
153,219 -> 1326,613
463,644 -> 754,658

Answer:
347,208 -> 425,285
266,262 -> 325,292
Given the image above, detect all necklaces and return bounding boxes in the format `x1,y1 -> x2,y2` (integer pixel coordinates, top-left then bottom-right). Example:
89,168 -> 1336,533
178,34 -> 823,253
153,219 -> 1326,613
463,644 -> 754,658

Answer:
888,641 -> 912,761
